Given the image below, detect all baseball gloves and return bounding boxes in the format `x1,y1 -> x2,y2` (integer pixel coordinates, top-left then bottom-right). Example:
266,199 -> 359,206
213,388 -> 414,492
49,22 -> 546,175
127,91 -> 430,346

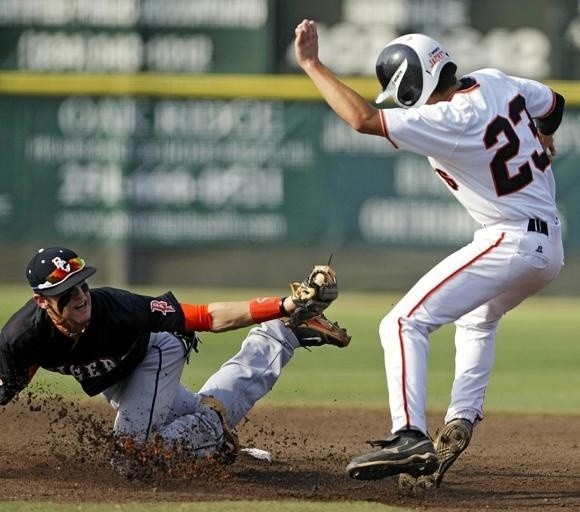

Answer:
284,265 -> 339,327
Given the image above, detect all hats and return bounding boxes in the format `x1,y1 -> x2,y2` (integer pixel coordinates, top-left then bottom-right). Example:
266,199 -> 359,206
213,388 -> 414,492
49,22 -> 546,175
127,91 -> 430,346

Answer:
26,246 -> 98,298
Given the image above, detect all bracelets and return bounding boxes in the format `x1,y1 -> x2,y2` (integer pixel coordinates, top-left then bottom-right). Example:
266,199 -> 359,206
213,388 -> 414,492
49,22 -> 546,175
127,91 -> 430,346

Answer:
249,295 -> 288,324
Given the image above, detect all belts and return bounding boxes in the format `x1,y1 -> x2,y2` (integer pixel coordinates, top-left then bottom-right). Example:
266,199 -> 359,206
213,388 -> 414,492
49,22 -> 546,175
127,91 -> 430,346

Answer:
528,217 -> 549,237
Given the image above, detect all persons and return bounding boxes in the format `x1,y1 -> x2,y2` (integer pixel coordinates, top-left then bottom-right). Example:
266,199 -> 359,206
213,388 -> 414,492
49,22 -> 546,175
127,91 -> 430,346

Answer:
1,247 -> 351,476
294,20 -> 565,495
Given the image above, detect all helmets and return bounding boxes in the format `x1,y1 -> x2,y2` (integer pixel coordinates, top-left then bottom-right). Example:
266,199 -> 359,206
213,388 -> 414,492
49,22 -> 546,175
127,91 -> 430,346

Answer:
375,32 -> 457,110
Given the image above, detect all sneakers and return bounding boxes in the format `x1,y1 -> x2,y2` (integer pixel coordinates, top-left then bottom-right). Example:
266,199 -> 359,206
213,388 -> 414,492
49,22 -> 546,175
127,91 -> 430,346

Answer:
201,396 -> 239,465
282,314 -> 353,347
397,419 -> 474,491
346,428 -> 440,481
